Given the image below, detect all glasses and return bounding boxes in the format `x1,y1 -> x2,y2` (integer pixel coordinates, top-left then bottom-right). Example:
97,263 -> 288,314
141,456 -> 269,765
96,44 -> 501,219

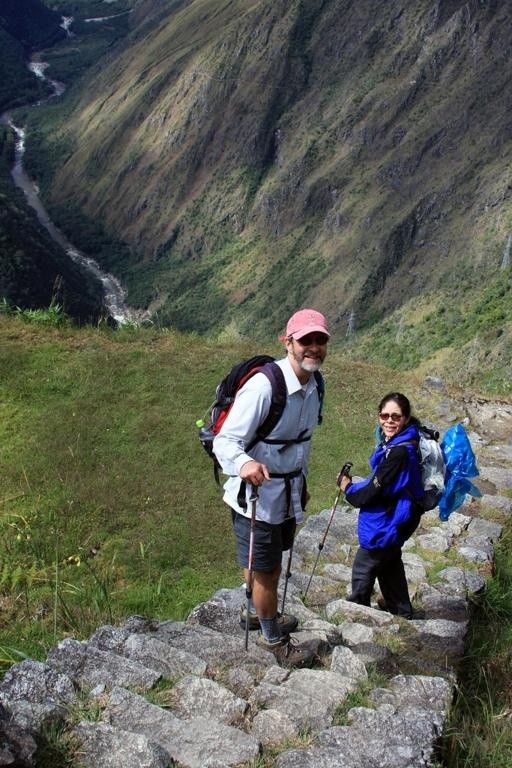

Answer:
294,336 -> 330,347
378,412 -> 402,423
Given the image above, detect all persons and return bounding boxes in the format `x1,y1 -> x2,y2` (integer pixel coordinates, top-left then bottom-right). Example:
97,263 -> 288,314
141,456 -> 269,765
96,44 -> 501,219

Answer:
335,391 -> 426,619
211,309 -> 332,667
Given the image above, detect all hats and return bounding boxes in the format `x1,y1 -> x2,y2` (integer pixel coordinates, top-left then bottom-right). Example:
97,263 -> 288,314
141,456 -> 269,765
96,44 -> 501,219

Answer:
283,308 -> 332,340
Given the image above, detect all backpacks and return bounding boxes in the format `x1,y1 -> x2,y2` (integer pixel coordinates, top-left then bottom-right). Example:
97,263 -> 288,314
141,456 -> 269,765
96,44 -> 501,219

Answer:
416,425 -> 448,513
196,353 -> 325,471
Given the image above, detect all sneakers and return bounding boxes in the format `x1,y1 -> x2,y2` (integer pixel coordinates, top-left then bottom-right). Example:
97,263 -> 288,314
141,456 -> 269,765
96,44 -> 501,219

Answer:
254,631 -> 319,670
237,604 -> 299,633
376,597 -> 415,619
